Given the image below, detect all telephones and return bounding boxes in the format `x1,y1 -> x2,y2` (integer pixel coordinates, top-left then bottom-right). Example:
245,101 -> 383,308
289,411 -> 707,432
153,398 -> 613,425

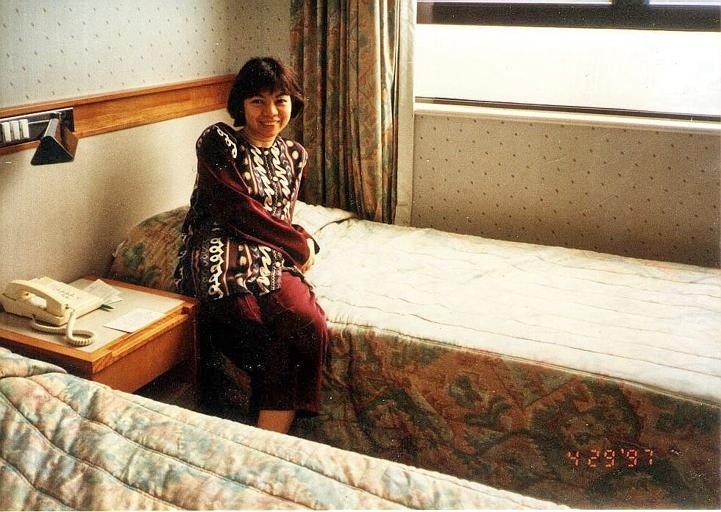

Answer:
1,275 -> 103,346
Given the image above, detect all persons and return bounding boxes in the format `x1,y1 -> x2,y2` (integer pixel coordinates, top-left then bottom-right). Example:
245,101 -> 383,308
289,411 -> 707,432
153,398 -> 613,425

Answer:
171,55 -> 330,435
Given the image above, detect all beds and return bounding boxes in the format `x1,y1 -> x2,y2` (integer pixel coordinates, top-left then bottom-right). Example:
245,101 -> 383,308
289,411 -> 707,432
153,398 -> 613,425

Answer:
0,346 -> 568,510
100,199 -> 720,509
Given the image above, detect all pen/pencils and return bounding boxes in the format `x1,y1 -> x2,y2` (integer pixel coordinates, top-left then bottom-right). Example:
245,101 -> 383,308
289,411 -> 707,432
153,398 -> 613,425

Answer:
101,304 -> 114,309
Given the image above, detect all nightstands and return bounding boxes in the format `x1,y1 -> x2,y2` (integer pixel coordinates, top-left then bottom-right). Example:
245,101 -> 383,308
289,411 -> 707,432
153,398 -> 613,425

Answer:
1,273 -> 200,406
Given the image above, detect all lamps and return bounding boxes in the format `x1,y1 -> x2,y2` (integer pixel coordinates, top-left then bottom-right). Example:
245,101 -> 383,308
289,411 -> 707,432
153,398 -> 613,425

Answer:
3,107 -> 84,166
